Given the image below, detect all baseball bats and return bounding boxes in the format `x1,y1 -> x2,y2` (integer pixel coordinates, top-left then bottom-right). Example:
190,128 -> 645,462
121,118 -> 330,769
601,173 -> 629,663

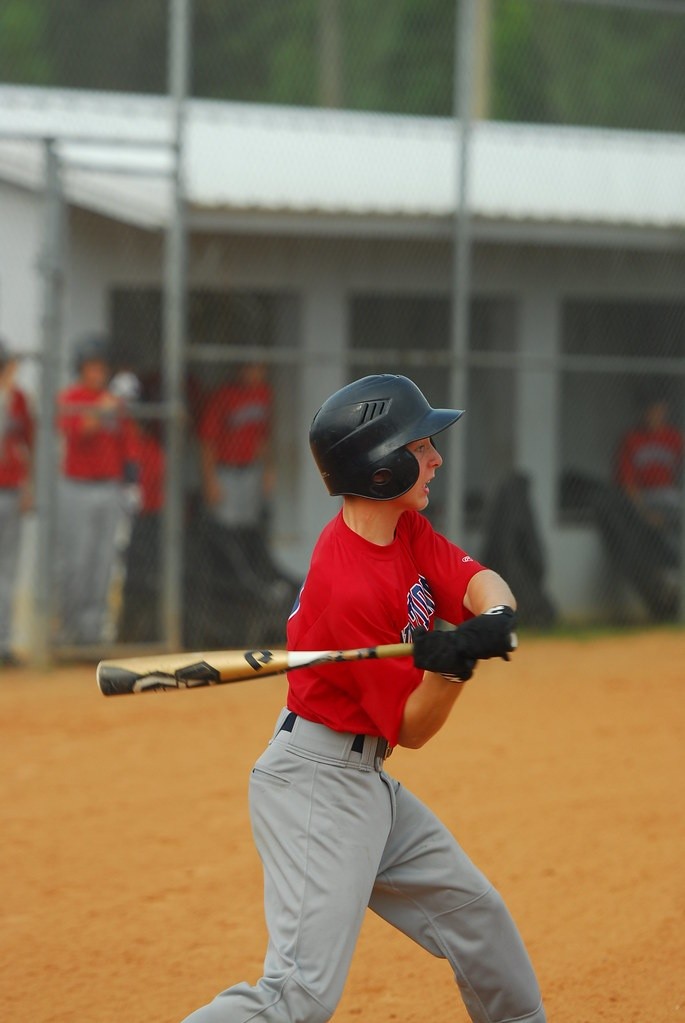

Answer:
95,631 -> 519,703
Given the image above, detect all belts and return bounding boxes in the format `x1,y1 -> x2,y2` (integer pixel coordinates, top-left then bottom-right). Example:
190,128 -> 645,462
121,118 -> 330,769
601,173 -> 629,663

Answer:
282,709 -> 392,762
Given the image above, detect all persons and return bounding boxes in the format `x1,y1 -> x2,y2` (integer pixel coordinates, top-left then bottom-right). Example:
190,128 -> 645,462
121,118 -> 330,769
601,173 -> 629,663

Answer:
181,377 -> 548,1023
1,339 -> 38,669
617,397 -> 685,567
38,336 -> 149,658
192,355 -> 279,655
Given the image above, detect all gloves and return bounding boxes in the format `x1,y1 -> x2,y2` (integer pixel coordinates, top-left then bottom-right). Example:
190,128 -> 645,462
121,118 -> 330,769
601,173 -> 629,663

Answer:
409,627 -> 479,686
458,604 -> 520,664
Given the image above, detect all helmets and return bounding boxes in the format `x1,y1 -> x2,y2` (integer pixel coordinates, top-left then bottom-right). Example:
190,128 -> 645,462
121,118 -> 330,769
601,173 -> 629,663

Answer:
308,373 -> 466,503
